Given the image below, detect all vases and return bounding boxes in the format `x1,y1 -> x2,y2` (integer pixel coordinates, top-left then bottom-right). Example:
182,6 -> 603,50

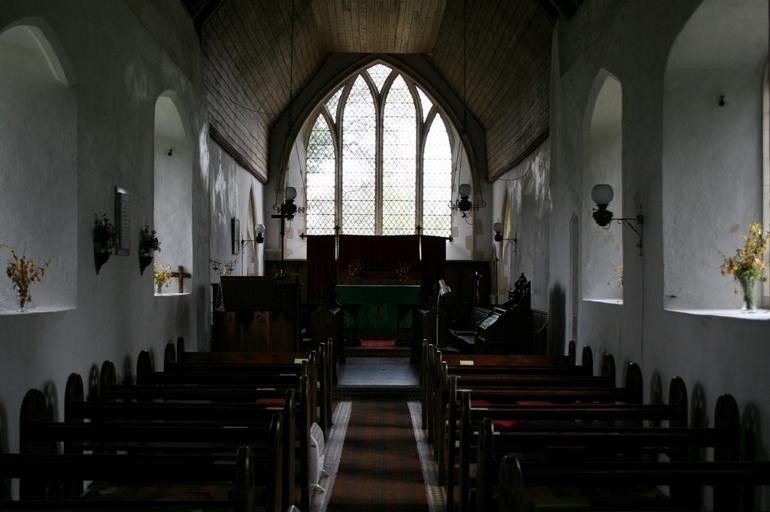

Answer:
15,294 -> 25,311
741,279 -> 757,313
156,283 -> 163,294
95,251 -> 108,274
140,256 -> 152,275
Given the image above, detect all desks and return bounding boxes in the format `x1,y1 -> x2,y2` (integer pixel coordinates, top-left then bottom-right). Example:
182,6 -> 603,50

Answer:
335,283 -> 422,364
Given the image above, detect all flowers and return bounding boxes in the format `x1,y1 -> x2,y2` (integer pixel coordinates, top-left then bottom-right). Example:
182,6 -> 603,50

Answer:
153,270 -> 173,288
0,242 -> 49,300
137,224 -> 162,255
93,211 -> 119,256
716,217 -> 770,294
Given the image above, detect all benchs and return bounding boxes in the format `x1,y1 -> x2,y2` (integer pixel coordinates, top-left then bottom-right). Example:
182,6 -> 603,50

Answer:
417,337 -> 770,512
0,334 -> 333,512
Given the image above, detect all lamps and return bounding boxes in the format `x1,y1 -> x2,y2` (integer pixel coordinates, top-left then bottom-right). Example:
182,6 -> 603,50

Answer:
494,223 -> 517,252
241,223 -> 265,246
273,185 -> 305,215
449,184 -> 485,218
435,278 -> 452,346
591,183 -> 644,255
